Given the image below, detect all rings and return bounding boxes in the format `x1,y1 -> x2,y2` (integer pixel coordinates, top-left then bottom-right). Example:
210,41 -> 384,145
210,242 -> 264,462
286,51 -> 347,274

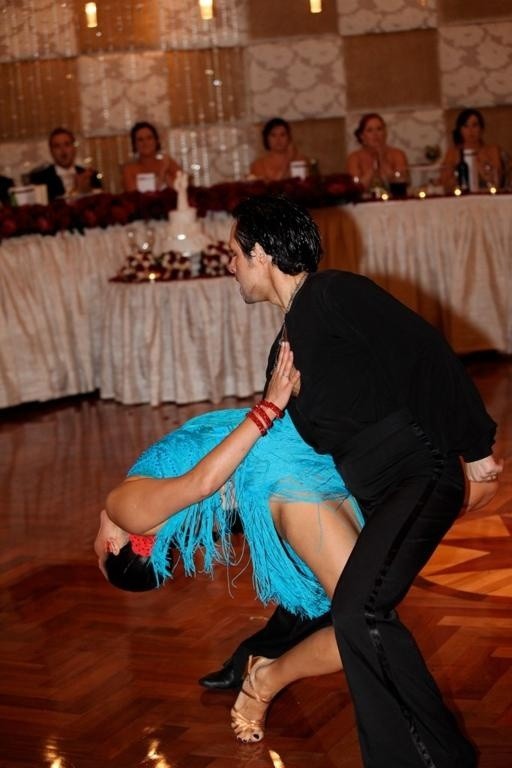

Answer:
282,373 -> 288,377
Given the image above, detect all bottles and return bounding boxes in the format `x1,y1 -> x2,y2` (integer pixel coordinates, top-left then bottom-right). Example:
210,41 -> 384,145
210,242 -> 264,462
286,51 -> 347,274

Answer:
456,146 -> 471,194
367,155 -> 385,200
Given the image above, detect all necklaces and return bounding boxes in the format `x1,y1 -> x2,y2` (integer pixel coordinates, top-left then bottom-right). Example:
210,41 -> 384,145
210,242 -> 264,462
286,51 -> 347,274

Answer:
269,273 -> 307,374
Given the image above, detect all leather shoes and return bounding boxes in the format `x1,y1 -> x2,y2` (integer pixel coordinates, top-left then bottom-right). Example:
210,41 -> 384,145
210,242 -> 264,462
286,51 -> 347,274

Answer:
200,665 -> 242,690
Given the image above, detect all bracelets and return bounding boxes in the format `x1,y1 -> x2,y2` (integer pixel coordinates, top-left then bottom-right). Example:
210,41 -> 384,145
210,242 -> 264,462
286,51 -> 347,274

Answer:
259,399 -> 284,418
246,411 -> 267,437
252,405 -> 274,429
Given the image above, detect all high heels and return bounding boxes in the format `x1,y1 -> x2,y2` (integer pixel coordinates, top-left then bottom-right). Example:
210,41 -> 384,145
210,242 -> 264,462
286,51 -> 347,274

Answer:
229,653 -> 278,744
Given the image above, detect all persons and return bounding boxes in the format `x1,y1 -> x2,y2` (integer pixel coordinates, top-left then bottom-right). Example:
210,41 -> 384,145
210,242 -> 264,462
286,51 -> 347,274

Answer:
249,117 -> 307,184
196,178 -> 505,767
435,107 -> 502,193
93,339 -> 365,742
123,120 -> 193,193
344,112 -> 408,199
29,125 -> 104,203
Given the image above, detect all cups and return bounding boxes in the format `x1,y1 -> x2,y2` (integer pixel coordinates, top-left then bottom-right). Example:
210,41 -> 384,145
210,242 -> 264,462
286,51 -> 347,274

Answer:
113,208 -> 233,282
388,169 -> 411,198
